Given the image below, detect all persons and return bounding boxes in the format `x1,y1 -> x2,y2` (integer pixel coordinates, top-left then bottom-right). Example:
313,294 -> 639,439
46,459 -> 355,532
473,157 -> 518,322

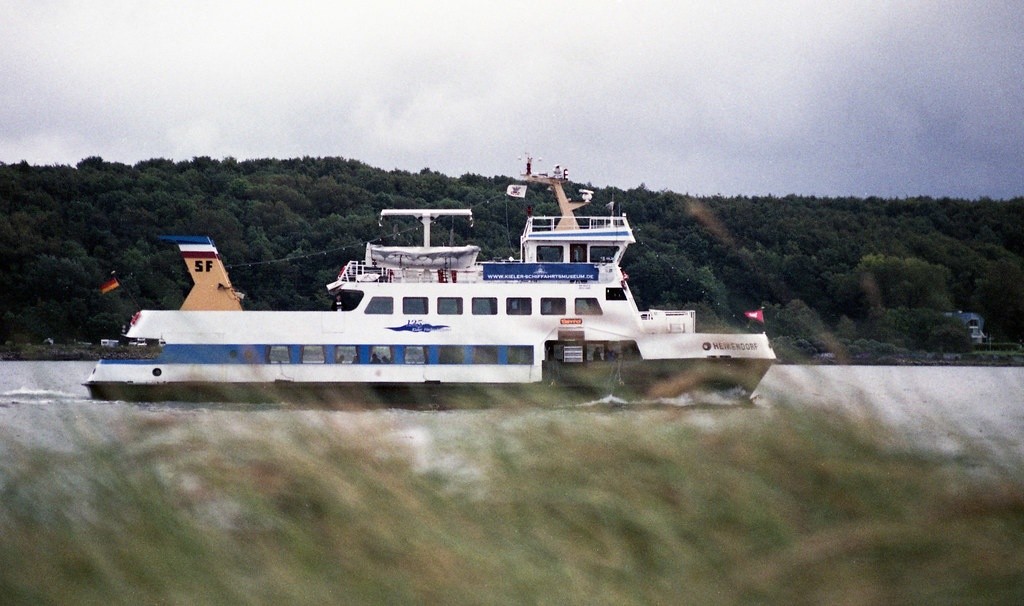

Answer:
593,347 -> 603,361
352,354 -> 360,364
370,353 -> 391,363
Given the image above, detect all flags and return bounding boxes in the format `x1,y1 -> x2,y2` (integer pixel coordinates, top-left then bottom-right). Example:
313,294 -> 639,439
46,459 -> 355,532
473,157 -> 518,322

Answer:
101,276 -> 120,294
506,184 -> 527,199
744,310 -> 764,323
605,201 -> 615,210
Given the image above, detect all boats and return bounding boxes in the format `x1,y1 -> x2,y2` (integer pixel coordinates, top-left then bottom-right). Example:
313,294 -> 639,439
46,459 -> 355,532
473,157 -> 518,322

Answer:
81,147 -> 777,410
